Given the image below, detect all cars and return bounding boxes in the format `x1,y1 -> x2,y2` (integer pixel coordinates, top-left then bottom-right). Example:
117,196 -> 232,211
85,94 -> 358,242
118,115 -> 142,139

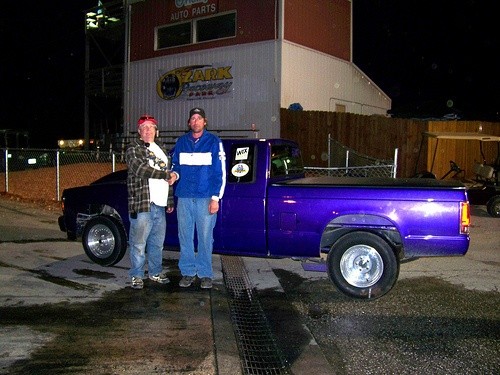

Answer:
16,147 -> 56,172
408,160 -> 500,216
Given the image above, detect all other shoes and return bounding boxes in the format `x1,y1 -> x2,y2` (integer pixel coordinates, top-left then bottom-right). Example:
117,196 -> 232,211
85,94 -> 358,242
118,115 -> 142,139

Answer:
131,276 -> 143,289
148,273 -> 170,284
200,277 -> 212,288
179,276 -> 196,287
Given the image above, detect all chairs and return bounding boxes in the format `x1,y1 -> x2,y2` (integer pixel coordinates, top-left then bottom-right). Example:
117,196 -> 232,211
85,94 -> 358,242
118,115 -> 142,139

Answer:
457,163 -> 493,189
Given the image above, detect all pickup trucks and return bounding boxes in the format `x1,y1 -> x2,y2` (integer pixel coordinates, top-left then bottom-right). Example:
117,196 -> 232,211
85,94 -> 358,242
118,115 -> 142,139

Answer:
59,136 -> 470,298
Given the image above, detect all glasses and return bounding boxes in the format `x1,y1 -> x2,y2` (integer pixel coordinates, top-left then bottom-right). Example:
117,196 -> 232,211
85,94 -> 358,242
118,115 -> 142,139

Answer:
140,117 -> 154,120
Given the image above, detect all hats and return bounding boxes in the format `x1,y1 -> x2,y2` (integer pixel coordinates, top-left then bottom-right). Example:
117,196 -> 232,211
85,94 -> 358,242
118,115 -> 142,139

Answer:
138,114 -> 157,125
189,107 -> 205,118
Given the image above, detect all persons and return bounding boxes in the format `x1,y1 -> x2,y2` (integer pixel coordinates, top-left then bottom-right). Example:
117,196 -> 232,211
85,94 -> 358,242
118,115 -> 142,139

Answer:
124,113 -> 174,289
170,106 -> 228,289
